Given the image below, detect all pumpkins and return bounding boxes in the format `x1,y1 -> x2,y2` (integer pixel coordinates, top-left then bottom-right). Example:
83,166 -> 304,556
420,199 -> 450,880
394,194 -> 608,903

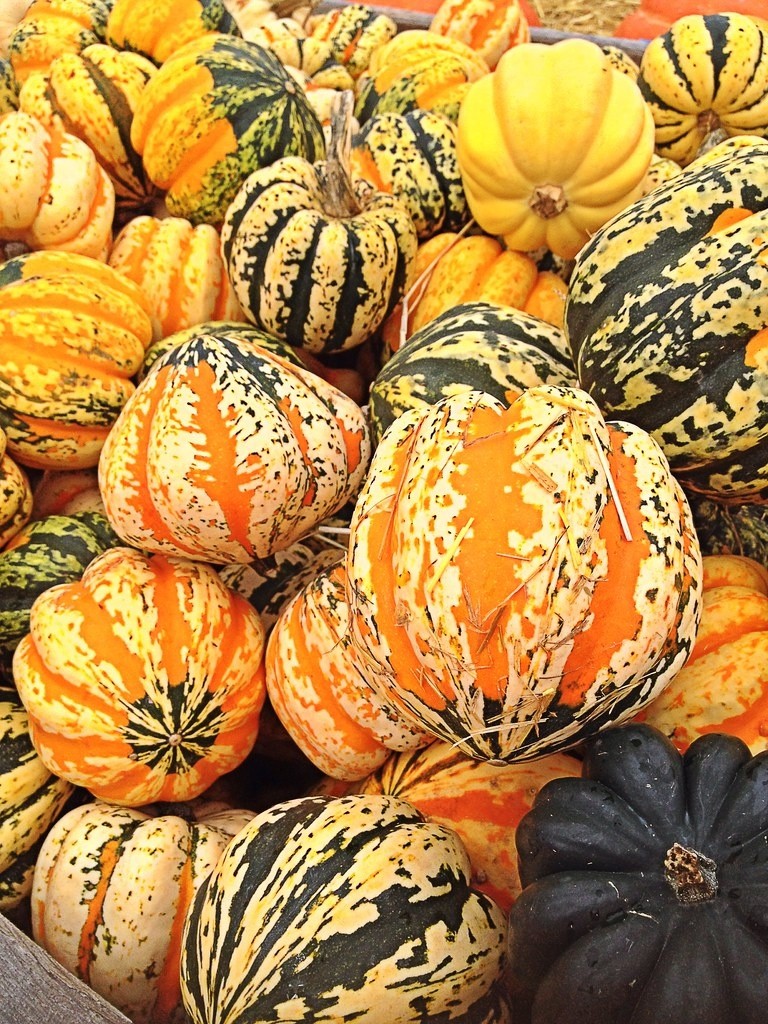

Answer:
0,0 -> 767,1024
